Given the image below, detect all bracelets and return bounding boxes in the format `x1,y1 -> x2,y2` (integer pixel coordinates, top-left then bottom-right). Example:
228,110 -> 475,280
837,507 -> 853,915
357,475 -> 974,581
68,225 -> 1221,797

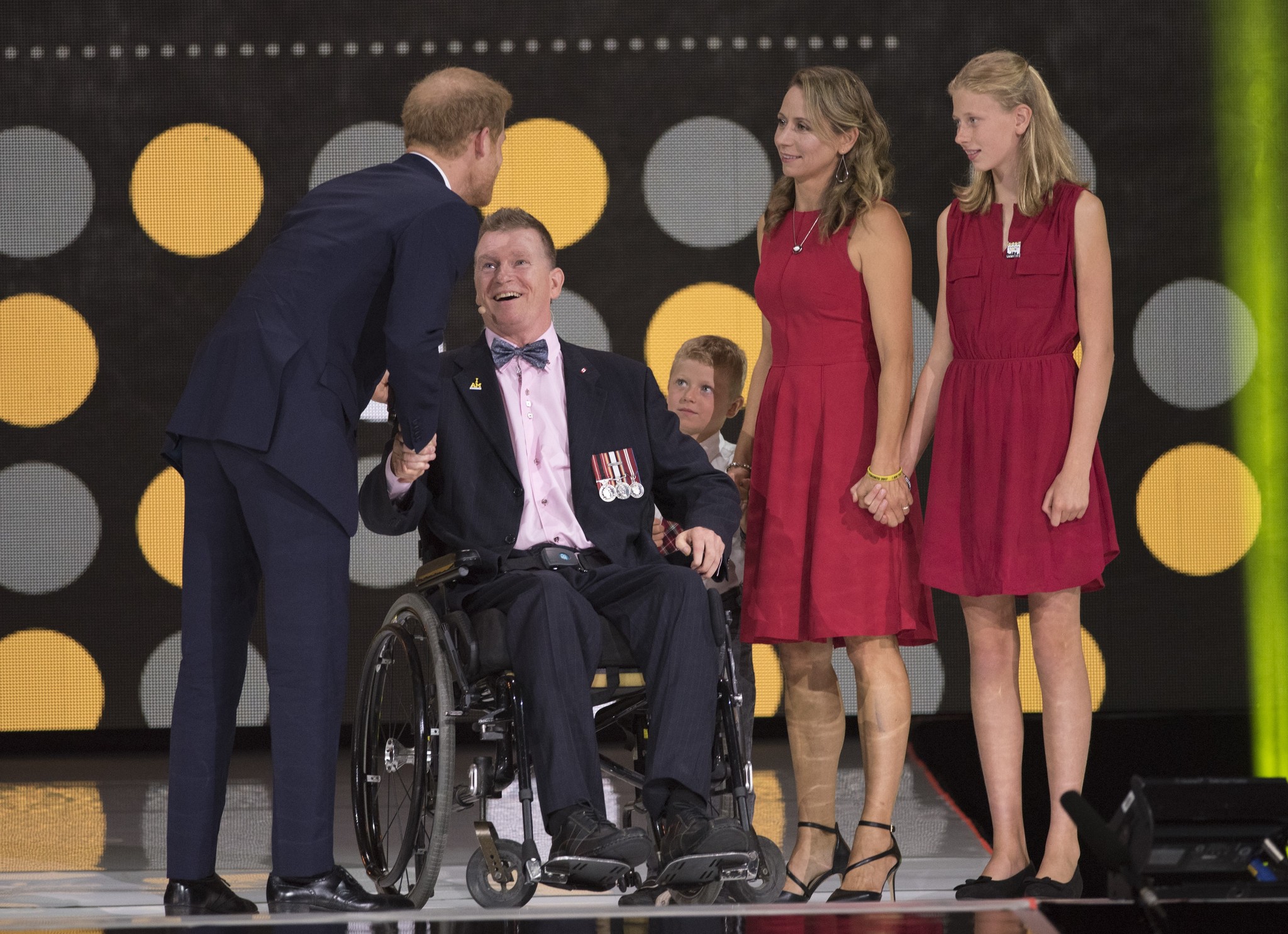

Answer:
726,462 -> 751,474
867,465 -> 903,482
901,473 -> 911,491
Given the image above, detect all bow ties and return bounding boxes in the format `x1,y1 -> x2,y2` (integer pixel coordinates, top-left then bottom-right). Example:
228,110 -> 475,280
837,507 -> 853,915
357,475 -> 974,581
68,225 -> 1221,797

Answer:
490,337 -> 548,370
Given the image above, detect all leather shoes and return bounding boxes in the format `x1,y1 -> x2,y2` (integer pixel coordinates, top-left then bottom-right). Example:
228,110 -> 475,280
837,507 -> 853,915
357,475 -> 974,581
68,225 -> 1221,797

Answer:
265,862 -> 417,913
163,871 -> 260,917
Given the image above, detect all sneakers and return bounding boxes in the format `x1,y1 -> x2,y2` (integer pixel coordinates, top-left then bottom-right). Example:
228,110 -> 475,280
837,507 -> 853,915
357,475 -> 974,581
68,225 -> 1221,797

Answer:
661,813 -> 749,872
548,811 -> 653,869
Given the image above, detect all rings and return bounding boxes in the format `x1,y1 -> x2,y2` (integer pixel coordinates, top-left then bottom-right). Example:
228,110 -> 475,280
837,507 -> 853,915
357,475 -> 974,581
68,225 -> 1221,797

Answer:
902,504 -> 910,510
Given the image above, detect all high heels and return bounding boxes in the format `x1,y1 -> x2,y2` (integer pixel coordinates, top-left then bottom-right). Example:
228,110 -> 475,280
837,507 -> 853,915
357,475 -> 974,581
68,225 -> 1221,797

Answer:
775,822 -> 852,903
826,820 -> 902,903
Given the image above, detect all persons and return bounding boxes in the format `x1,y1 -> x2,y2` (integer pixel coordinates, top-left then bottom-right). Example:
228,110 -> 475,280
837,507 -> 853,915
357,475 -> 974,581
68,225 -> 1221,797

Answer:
358,207 -> 741,879
617,334 -> 756,909
159,66 -> 513,918
848,49 -> 1117,900
724,64 -> 914,905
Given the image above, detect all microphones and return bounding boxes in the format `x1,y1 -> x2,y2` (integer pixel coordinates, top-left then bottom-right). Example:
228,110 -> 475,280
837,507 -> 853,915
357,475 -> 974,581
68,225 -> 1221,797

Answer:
478,307 -> 486,314
1061,790 -> 1165,921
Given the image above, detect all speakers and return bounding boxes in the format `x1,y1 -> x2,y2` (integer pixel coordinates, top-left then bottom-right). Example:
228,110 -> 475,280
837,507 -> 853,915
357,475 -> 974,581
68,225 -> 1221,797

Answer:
1106,778 -> 1288,891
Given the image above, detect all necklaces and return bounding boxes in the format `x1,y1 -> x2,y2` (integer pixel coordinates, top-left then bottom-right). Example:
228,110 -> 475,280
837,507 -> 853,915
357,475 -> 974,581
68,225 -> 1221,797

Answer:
791,201 -> 823,254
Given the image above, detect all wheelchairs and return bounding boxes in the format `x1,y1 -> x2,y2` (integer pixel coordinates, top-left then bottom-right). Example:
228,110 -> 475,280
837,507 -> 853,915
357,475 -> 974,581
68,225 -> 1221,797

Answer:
350,538 -> 787,907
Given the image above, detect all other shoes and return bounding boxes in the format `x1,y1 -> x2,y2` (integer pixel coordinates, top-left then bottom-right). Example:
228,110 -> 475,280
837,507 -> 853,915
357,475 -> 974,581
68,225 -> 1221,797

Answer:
1025,865 -> 1083,899
953,860 -> 1036,901
